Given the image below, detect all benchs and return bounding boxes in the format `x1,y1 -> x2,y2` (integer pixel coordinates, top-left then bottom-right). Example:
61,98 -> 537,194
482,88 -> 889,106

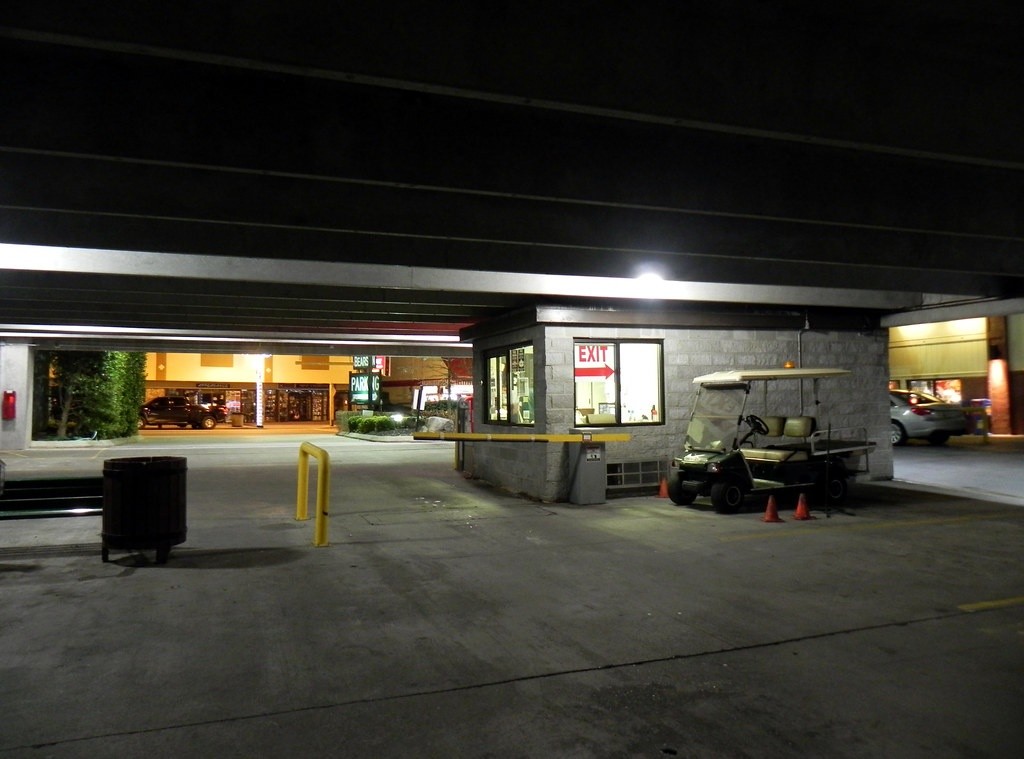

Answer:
739,415 -> 815,461
0,476 -> 105,520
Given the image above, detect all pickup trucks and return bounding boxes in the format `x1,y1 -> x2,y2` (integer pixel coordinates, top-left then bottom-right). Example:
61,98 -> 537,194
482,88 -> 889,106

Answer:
136,396 -> 228,427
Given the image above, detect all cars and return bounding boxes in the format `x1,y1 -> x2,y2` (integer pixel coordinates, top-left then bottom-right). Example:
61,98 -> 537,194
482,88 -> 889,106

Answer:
888,389 -> 967,448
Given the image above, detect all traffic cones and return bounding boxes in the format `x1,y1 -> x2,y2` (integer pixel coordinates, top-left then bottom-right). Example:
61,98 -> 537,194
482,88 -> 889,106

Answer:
760,496 -> 787,524
791,493 -> 817,520
656,478 -> 669,499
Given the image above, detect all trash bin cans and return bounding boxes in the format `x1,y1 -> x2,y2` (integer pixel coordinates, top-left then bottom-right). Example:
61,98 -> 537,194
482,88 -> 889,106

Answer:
970,399 -> 992,435
232,412 -> 243,427
455,396 -> 473,471
568,428 -> 606,505
101,456 -> 188,565
